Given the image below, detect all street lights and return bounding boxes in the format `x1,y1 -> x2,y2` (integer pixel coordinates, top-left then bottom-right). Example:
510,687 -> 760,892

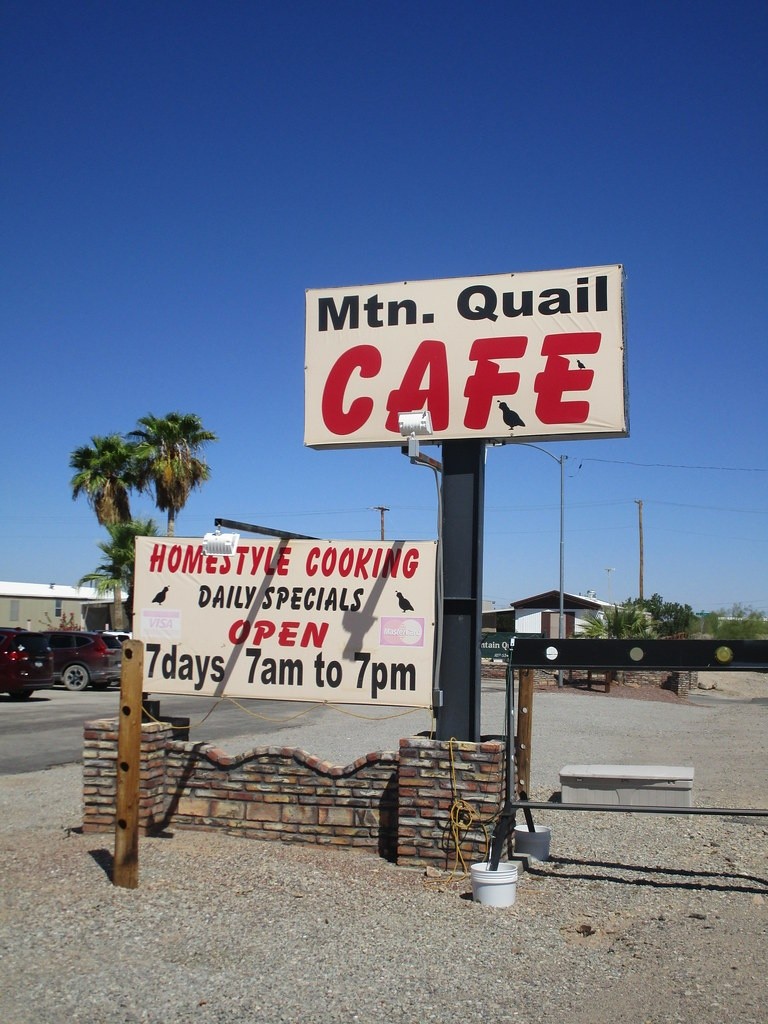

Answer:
493,442 -> 567,688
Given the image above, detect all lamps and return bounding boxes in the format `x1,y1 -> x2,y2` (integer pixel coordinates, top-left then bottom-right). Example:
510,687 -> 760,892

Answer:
398,410 -> 434,458
202,525 -> 240,558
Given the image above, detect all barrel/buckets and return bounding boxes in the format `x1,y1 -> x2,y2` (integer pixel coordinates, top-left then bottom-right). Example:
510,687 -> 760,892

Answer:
514,825 -> 551,861
470,863 -> 517,907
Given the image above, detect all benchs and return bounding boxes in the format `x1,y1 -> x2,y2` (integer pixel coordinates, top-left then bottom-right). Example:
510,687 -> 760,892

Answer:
561,766 -> 696,821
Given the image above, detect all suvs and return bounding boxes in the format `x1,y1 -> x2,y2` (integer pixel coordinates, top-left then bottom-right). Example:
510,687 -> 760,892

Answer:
99,629 -> 132,643
0,625 -> 55,699
41,631 -> 123,691
